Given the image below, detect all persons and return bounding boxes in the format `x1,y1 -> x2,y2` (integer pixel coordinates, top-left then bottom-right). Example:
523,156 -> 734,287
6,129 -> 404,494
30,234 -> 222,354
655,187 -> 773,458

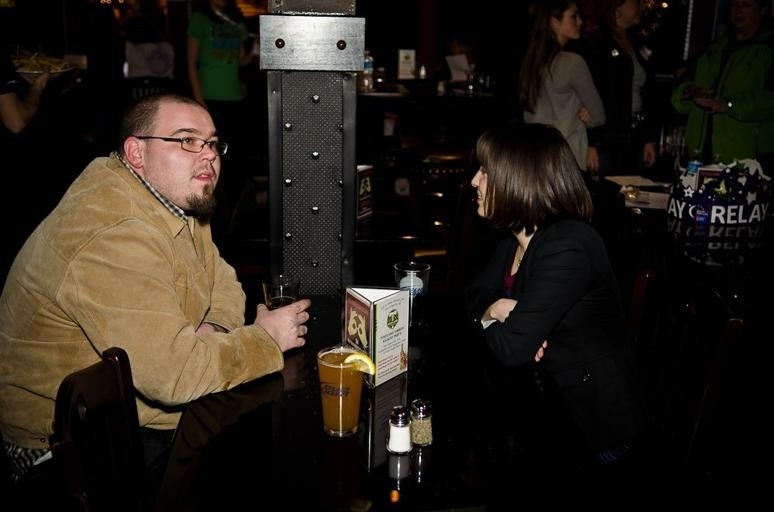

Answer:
186,0 -> 259,143
0,1 -> 70,136
670,1 -> 773,170
412,123 -> 626,366
0,91 -> 312,482
588,0 -> 660,175
519,0 -> 607,177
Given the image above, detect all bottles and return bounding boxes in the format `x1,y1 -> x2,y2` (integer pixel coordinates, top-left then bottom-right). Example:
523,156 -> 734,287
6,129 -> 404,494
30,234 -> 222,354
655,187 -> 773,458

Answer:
385,403 -> 414,456
409,398 -> 433,447
357,51 -> 373,93
686,149 -> 704,177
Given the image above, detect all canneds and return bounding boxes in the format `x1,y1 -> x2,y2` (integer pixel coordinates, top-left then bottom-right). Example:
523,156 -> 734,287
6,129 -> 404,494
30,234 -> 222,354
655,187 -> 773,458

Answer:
686,160 -> 703,176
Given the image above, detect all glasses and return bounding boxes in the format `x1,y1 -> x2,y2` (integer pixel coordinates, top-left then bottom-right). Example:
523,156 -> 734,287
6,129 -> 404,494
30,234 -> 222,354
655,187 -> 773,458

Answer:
138,135 -> 230,156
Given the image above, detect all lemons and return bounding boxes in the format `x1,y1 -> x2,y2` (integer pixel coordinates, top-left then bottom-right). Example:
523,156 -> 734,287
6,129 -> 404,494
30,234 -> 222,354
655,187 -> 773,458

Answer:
343,353 -> 375,375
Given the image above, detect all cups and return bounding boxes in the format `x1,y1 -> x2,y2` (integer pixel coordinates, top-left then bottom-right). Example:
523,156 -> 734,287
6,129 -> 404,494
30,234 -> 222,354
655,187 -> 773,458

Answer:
261,274 -> 301,349
392,261 -> 432,332
317,344 -> 366,439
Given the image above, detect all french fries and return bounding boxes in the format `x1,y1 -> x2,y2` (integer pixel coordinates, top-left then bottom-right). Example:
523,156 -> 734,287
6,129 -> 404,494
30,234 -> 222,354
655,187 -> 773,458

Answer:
13,49 -> 71,72
